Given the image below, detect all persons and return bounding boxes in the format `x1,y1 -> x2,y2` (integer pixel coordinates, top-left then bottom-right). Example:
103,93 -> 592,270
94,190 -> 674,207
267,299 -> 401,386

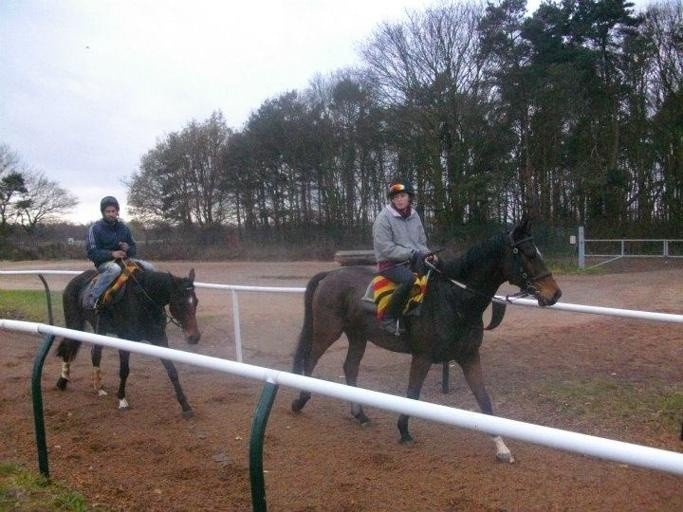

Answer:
371,179 -> 442,338
83,195 -> 156,317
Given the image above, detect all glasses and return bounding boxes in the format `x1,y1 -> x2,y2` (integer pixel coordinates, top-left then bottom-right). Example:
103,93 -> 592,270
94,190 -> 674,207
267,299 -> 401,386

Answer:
101,198 -> 116,204
389,184 -> 406,194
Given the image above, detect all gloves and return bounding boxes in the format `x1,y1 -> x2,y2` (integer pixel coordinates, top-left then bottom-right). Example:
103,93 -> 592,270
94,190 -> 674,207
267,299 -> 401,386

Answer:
413,251 -> 428,261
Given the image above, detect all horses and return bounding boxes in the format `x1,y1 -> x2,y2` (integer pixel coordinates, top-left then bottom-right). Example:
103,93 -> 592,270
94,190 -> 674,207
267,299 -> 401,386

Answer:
290,221 -> 561,463
54,268 -> 200,418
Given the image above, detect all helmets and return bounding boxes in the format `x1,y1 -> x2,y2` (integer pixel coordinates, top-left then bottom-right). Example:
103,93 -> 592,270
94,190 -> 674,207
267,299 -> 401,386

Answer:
100,196 -> 119,212
388,179 -> 415,196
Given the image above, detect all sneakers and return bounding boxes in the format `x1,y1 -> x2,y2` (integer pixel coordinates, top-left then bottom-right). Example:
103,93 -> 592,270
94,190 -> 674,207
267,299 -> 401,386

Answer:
380,316 -> 407,337
83,290 -> 98,312
